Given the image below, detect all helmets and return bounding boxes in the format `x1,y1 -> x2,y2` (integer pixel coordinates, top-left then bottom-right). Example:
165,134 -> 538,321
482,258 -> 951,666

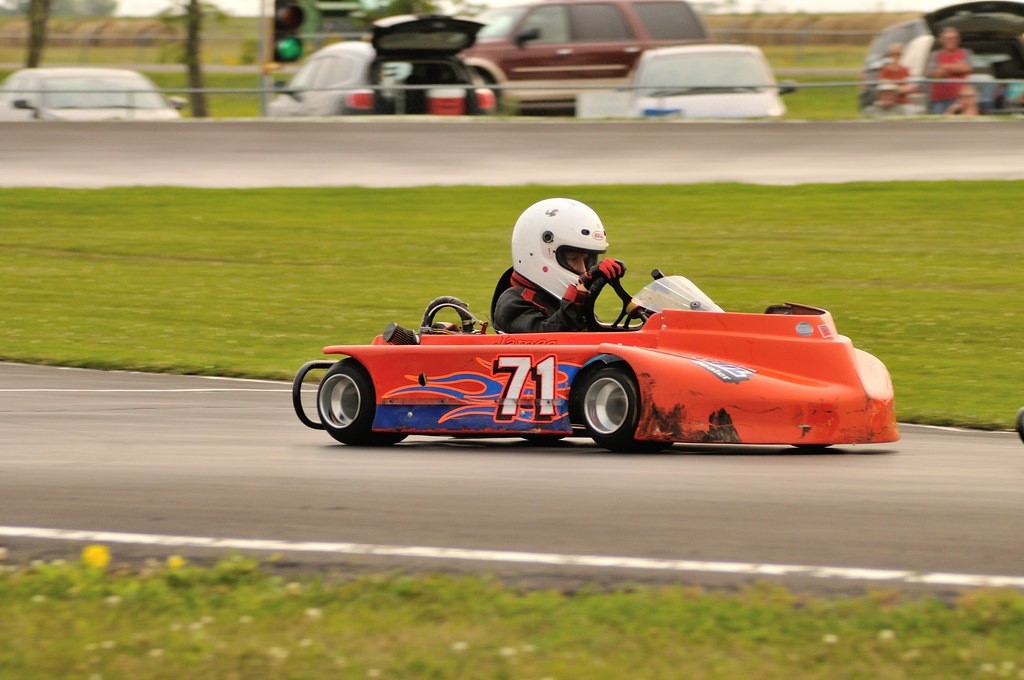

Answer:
511,197 -> 609,300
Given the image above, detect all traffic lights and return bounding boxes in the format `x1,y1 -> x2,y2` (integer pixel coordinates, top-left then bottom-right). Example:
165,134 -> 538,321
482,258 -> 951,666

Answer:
272,4 -> 306,32
275,39 -> 305,64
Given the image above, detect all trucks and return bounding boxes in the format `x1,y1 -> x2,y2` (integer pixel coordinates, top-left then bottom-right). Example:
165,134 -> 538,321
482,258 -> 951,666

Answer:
573,40 -> 803,130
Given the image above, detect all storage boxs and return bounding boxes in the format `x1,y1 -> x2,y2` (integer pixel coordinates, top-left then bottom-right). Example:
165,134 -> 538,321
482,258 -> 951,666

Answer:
427,88 -> 468,114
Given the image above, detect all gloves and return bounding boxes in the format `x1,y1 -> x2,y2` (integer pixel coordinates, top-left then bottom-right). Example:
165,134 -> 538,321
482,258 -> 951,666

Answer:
578,257 -> 627,292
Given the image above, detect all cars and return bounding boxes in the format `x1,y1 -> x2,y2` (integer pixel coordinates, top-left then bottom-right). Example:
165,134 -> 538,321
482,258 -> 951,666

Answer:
866,2 -> 1023,115
3,60 -> 188,122
264,13 -> 513,113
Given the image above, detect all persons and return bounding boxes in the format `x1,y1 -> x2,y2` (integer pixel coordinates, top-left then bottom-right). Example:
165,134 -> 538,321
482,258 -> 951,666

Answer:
945,86 -> 985,115
878,42 -> 911,106
494,197 -> 641,332
924,27 -> 974,113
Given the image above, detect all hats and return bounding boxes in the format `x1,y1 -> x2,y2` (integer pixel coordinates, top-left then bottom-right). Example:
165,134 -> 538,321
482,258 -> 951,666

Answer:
889,42 -> 903,55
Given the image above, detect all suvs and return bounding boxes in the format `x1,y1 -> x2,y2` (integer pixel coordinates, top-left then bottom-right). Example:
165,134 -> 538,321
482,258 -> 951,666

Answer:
462,0 -> 710,113
